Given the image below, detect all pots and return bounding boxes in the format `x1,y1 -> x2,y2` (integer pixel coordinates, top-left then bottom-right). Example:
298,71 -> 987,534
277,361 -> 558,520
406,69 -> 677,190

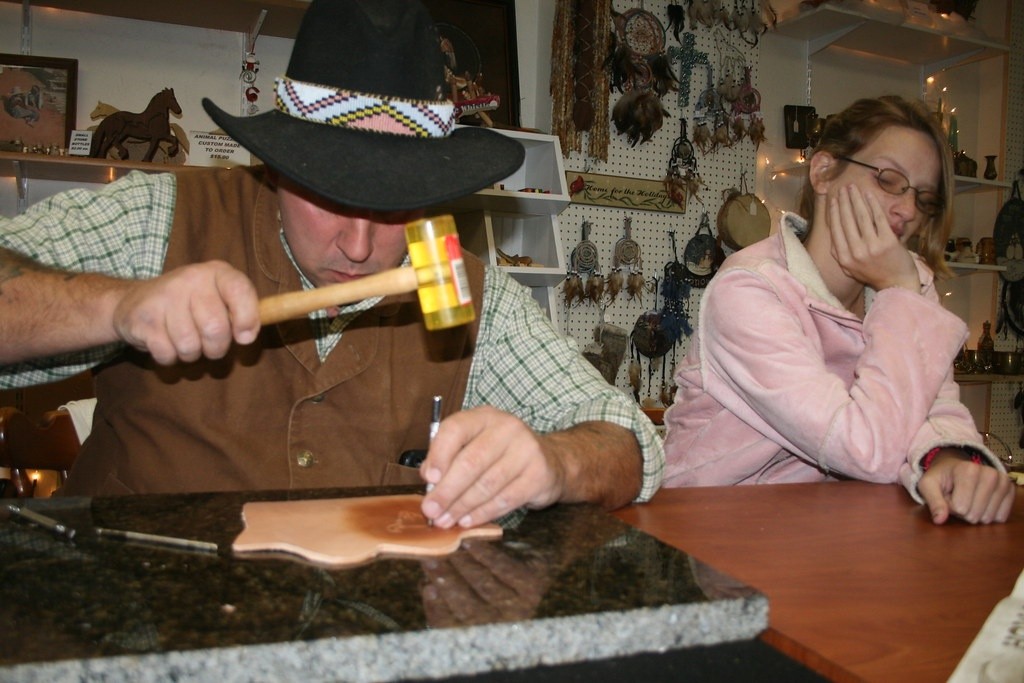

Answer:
989,347 -> 1024,375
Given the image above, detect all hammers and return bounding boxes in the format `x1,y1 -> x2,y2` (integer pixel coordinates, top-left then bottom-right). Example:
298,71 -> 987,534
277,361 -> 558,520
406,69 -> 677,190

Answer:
258,214 -> 479,336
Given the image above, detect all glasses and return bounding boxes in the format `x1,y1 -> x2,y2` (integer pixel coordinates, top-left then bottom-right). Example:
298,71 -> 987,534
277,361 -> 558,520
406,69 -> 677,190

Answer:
835,156 -> 947,218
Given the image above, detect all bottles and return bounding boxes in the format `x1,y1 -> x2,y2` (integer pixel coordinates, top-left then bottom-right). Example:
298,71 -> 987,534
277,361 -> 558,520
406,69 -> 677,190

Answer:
978,321 -> 993,369
984,155 -> 999,180
944,236 -> 995,265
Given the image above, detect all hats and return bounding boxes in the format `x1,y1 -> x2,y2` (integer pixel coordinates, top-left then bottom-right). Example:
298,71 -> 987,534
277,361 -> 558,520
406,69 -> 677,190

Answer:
203,0 -> 525,212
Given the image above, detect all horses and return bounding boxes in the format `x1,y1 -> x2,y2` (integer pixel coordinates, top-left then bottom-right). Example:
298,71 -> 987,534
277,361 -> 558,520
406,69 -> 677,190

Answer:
89,87 -> 182,163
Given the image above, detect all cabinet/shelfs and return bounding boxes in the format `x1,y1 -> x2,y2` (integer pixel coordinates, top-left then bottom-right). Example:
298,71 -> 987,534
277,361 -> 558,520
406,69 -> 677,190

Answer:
754,5 -> 1024,445
453,124 -> 571,333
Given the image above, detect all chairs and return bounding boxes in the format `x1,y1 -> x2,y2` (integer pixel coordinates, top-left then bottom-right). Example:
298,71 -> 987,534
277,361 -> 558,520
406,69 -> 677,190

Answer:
0,406 -> 81,499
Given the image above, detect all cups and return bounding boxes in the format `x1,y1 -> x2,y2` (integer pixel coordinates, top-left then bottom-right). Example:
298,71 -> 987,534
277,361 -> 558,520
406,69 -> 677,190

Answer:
965,349 -> 987,370
805,114 -> 839,147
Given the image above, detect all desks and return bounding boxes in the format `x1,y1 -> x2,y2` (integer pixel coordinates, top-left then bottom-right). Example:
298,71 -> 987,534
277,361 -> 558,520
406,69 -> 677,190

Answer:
615,480 -> 1024,683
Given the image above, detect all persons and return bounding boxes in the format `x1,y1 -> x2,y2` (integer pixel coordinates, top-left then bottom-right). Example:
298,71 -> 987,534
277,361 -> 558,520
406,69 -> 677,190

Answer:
1,0 -> 668,529
661,94 -> 1018,524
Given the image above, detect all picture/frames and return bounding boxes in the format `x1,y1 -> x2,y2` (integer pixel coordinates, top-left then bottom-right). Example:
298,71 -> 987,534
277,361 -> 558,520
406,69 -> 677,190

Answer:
0,53 -> 78,156
